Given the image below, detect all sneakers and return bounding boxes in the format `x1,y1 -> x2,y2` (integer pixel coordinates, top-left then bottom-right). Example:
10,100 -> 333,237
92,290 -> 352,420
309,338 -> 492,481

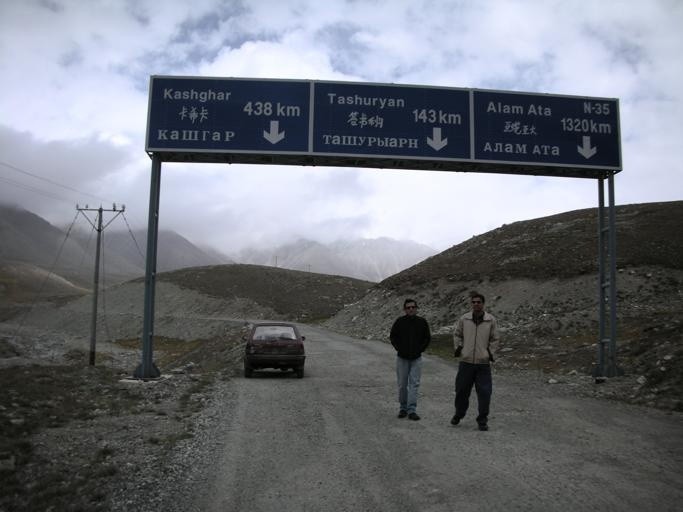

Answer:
398,410 -> 407,418
451,415 -> 460,425
479,423 -> 489,431
408,412 -> 420,420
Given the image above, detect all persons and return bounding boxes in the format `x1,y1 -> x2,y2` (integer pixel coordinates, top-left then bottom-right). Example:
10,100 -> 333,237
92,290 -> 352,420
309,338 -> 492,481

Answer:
451,294 -> 500,430
390,298 -> 430,420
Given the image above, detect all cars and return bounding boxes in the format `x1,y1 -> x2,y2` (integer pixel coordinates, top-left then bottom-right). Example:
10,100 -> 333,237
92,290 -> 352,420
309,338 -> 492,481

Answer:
242,321 -> 306,377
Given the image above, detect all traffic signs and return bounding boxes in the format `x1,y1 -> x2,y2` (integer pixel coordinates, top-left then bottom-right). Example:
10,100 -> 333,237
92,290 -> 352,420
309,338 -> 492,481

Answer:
146,76 -> 623,173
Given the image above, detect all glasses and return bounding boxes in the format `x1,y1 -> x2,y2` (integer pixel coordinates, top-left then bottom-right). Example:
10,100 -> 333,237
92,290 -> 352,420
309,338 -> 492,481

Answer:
407,306 -> 414,309
472,301 -> 480,304
